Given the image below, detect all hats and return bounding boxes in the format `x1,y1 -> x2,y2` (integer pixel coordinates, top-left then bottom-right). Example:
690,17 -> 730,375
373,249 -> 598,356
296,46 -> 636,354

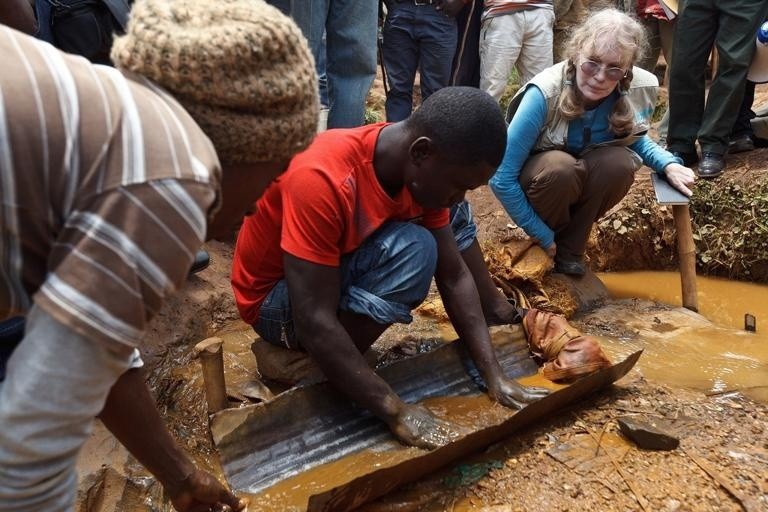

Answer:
107,3 -> 322,161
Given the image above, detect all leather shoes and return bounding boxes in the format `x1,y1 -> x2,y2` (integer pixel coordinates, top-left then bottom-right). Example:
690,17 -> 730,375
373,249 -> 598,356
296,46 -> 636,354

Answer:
555,249 -> 587,276
728,132 -> 755,154
669,145 -> 731,176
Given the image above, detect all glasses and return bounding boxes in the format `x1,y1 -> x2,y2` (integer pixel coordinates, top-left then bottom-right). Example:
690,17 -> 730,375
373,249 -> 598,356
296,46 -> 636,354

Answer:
581,59 -> 631,79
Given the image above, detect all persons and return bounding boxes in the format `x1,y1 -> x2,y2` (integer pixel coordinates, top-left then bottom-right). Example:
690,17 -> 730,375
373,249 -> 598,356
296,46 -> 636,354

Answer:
1,1 -> 768,279
225,83 -> 551,452
0,0 -> 322,512
